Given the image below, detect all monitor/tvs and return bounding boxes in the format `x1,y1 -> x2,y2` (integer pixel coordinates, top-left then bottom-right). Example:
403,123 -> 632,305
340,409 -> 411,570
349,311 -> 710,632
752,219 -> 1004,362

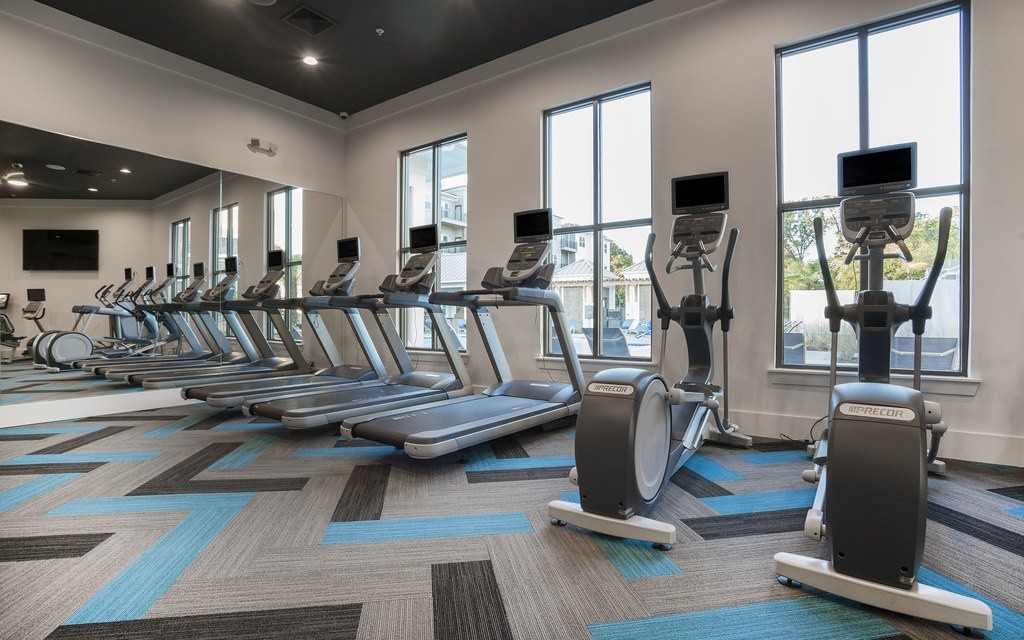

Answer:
512,207 -> 554,242
146,266 -> 154,279
409,224 -> 439,254
23,230 -> 99,270
167,264 -> 174,277
193,262 -> 205,279
336,237 -> 361,262
0,293 -> 10,308
267,250 -> 287,270
27,289 -> 45,301
125,268 -> 132,280
226,257 -> 237,274
837,141 -> 918,197
671,170 -> 729,214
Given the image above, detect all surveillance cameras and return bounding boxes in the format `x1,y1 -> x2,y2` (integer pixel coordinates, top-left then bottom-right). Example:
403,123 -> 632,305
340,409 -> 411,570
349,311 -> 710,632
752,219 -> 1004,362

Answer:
340,112 -> 349,120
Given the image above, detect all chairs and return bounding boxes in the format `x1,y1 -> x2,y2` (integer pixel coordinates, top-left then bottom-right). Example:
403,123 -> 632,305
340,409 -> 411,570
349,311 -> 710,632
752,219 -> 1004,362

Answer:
446,318 -> 466,349
891,337 -> 958,370
581,319 -> 651,357
784,332 -> 805,364
551,319 -> 578,353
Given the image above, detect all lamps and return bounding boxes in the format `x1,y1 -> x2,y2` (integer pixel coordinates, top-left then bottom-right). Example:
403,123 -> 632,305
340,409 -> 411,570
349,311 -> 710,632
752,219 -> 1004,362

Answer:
2,163 -> 27,187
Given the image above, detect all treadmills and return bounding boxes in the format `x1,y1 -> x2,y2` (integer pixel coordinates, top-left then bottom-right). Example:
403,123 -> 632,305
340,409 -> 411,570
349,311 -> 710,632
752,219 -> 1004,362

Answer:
180,236 -> 391,420
341,208 -> 586,460
71,249 -> 314,390
241,222 -> 474,430
